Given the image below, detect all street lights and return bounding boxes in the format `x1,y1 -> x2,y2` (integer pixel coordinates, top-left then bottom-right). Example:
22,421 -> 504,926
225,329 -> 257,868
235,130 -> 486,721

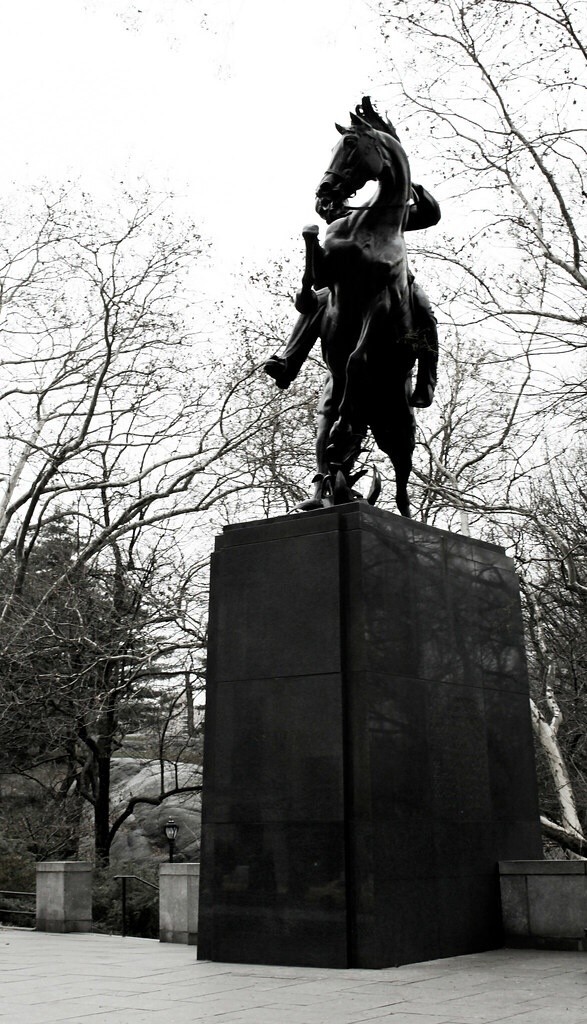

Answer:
164,817 -> 179,863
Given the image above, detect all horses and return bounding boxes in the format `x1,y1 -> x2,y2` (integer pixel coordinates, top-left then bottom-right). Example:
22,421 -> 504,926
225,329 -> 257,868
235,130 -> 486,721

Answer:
295,97 -> 442,516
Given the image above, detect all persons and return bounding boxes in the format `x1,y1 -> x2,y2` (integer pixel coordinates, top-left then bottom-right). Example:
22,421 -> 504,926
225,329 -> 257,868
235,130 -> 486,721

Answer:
264,195 -> 441,408
